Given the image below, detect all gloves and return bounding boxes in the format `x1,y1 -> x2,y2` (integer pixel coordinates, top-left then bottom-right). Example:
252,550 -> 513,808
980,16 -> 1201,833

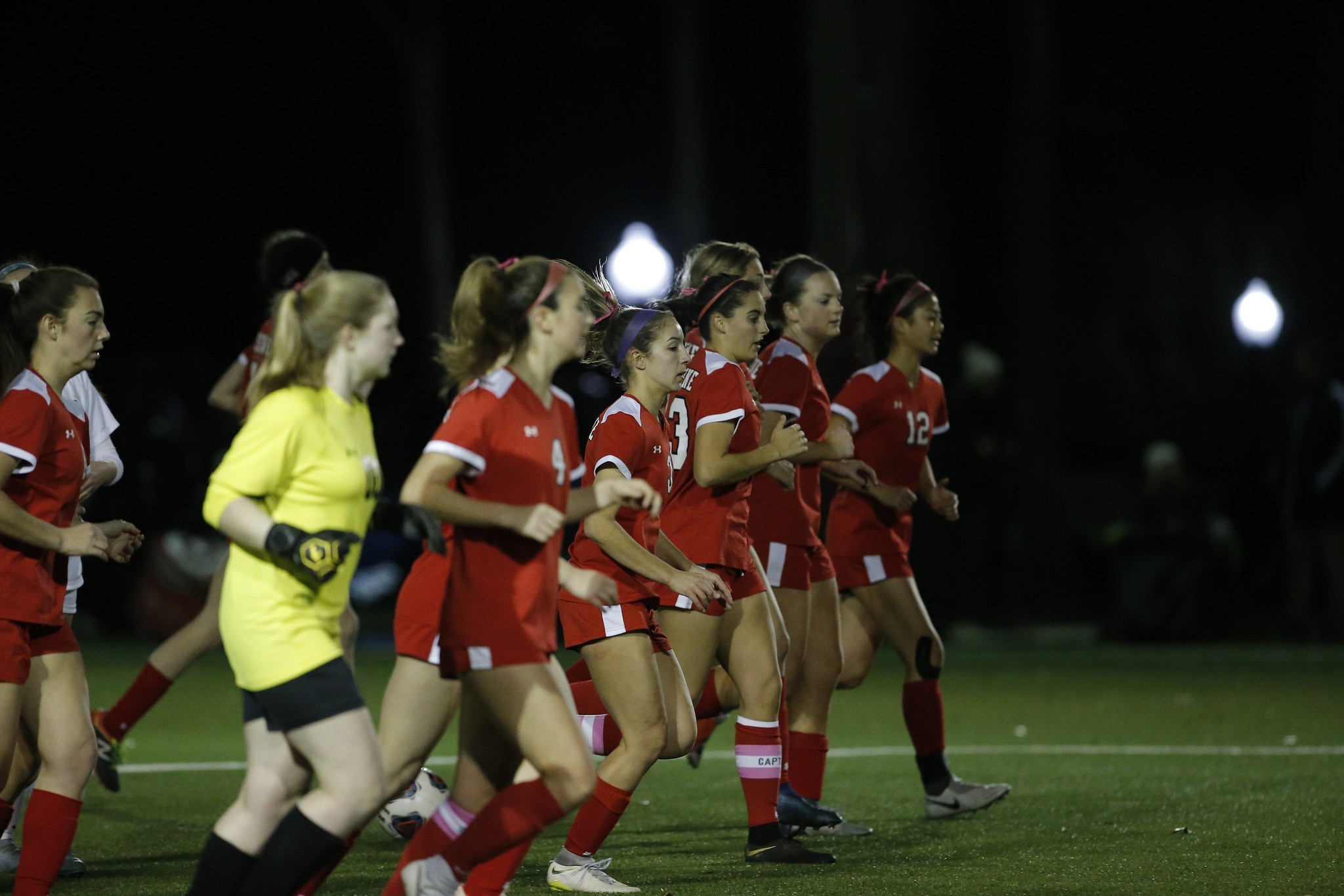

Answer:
265,523 -> 360,584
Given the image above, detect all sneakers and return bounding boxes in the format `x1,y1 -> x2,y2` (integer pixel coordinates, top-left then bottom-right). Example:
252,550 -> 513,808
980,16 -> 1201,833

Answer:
0,839 -> 22,872
687,714 -> 730,770
401,854 -> 466,896
57,848 -> 86,876
547,845 -> 642,894
745,837 -> 836,865
924,773 -> 1011,819
88,711 -> 121,792
791,806 -> 874,837
776,782 -> 842,830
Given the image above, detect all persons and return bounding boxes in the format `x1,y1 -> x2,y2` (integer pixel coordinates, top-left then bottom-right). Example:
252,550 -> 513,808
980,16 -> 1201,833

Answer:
685,268 -> 1013,821
644,239 -> 785,721
562,267 -> 835,862
90,229 -> 335,791
1103,434 -> 1245,633
183,269 -> 402,896
541,274 -> 735,896
695,243 -> 879,836
375,255 -> 622,896
1,266 -> 148,894
0,258 -> 131,878
296,249 -> 663,896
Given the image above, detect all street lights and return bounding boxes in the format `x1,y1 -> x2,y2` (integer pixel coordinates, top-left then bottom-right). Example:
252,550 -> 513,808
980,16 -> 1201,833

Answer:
1227,274 -> 1283,635
